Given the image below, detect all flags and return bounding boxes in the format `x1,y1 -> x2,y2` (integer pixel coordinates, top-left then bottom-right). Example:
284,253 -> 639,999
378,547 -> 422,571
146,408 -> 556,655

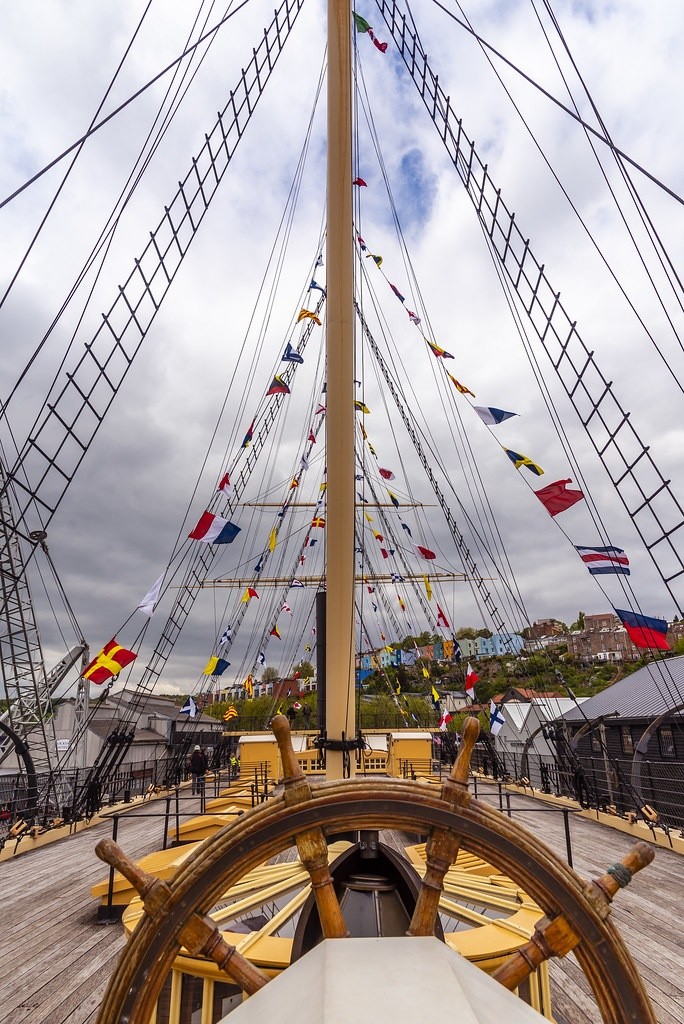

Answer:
533,478 -> 585,517
424,337 -> 456,359
489,700 -> 505,736
188,510 -> 241,544
575,546 -> 630,576
471,407 -> 519,424
354,221 -> 478,748
501,446 -> 544,476
352,176 -> 367,188
612,608 -> 672,651
353,12 -> 388,53
180,211 -> 327,722
138,572 -> 166,617
446,370 -> 476,397
80,638 -> 138,685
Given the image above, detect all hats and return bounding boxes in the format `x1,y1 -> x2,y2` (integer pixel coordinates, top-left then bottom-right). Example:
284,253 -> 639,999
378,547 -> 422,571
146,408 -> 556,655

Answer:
194,745 -> 200,751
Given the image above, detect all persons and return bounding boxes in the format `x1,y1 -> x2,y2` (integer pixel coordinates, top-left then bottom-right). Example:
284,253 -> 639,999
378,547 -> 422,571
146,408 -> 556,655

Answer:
286,705 -> 298,730
228,753 -> 240,776
303,702 -> 312,722
191,745 -> 209,794
261,688 -> 266,694
240,689 -> 248,702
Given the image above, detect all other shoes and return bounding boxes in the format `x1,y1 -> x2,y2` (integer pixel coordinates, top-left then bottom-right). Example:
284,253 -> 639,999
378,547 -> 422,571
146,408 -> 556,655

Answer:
192,792 -> 195,795
197,791 -> 201,794
